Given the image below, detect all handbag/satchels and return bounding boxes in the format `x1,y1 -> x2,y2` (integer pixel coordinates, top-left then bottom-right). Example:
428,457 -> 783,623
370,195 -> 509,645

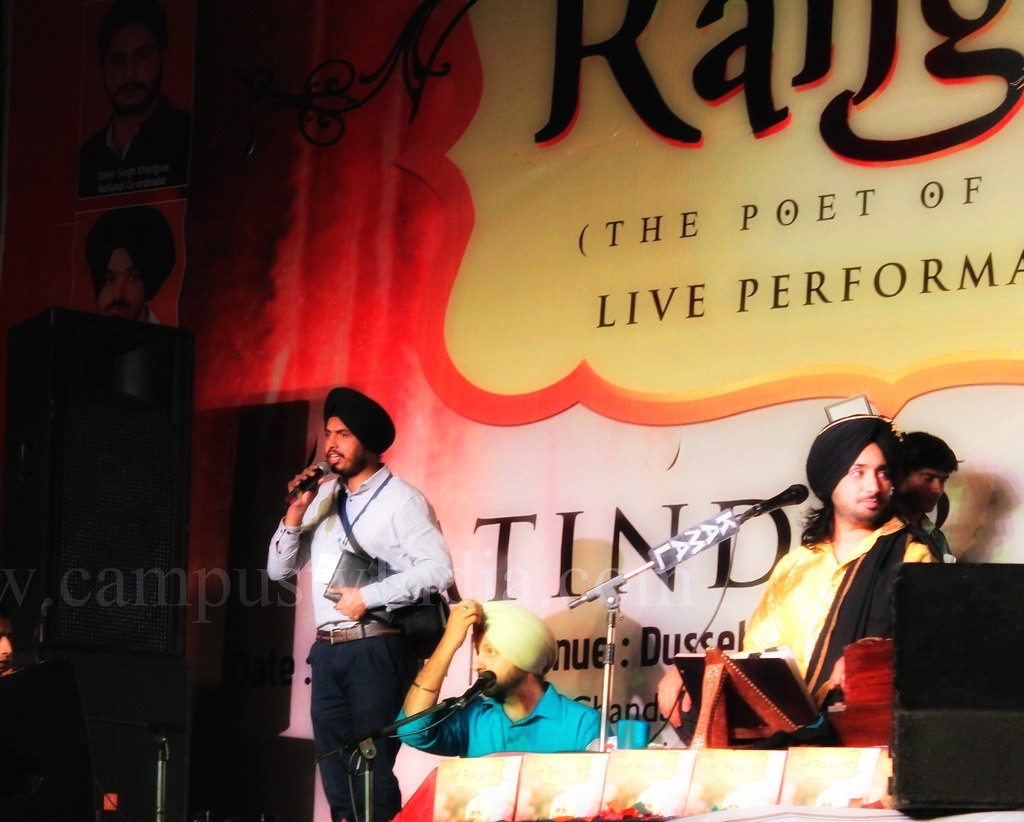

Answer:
402,592 -> 450,661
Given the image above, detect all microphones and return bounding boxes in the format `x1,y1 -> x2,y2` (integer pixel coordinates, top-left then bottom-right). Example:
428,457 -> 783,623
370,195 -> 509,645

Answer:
456,671 -> 496,709
735,484 -> 809,523
285,461 -> 330,504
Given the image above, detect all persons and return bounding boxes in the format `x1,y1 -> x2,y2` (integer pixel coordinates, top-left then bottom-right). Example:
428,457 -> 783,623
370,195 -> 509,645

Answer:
84,205 -> 174,324
0,604 -> 17,678
266,388 -> 455,822
893,430 -> 958,563
78,0 -> 191,198
657,414 -> 932,749
396,598 -> 615,755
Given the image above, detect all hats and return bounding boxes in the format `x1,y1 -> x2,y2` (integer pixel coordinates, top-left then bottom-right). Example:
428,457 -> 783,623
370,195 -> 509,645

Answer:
322,387 -> 396,456
805,415 -> 900,505
479,599 -> 559,676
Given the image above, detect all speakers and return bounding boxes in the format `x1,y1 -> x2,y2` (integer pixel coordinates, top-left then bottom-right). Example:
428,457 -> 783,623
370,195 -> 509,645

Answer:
889,562 -> 1024,819
0,304 -> 193,662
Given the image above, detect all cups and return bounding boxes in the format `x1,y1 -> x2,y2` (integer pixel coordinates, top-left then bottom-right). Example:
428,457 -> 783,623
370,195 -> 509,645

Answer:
615,718 -> 650,750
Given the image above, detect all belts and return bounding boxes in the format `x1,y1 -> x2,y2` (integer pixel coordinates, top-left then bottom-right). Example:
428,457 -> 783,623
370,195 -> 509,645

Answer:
316,622 -> 402,645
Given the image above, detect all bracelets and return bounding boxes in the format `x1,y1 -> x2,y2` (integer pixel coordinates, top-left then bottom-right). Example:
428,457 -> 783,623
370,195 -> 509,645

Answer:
414,681 -> 440,694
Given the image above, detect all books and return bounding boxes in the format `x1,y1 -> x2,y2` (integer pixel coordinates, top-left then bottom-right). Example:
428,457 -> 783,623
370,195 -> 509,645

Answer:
325,550 -> 399,619
673,645 -> 832,747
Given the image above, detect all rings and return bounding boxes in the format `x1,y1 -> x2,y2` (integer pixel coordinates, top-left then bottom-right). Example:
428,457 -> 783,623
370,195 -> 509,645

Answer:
464,606 -> 468,608
